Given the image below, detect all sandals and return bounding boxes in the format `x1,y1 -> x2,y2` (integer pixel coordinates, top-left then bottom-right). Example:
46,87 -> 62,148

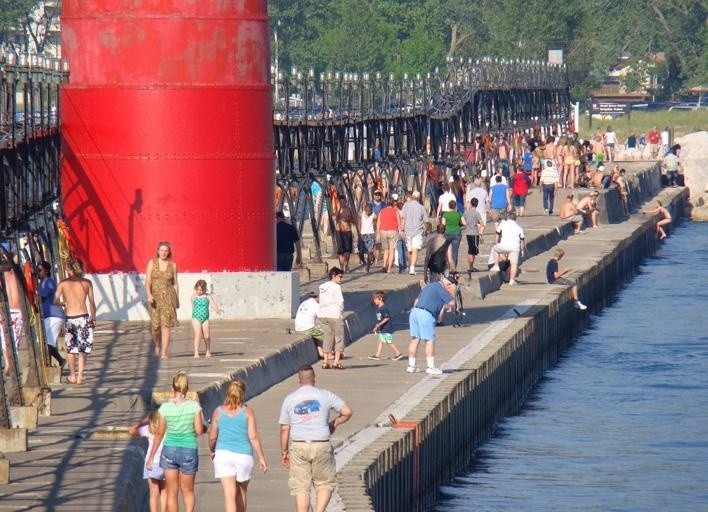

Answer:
322,362 -> 345,369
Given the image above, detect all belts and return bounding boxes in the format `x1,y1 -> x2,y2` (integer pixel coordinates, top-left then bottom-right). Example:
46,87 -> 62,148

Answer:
291,440 -> 330,443
416,306 -> 435,317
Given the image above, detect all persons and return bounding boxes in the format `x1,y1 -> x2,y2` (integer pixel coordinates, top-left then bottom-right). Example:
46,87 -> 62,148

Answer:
189,278 -> 224,360
274,108 -> 683,375
142,239 -> 181,360
277,364 -> 354,512
207,378 -> 269,512
128,372 -> 208,512
0,199 -> 98,385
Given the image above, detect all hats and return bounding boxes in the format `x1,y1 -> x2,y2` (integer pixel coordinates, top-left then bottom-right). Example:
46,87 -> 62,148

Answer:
39,260 -> 51,269
392,194 -> 398,200
374,190 -> 381,193
447,276 -> 458,285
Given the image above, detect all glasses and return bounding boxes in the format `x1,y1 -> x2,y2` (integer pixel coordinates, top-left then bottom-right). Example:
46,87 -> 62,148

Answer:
337,275 -> 342,278
374,194 -> 381,196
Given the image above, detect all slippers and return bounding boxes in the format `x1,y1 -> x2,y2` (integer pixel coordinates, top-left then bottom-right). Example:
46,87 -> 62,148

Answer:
468,268 -> 477,272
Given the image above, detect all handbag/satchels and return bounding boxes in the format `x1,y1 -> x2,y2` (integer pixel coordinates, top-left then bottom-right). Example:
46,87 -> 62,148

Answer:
428,239 -> 452,273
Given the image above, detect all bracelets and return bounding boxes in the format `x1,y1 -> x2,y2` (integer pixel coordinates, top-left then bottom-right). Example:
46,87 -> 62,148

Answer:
281,449 -> 288,454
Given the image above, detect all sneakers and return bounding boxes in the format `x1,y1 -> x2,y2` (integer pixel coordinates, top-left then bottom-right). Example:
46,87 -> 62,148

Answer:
392,354 -> 403,360
409,271 -> 417,275
489,266 -> 500,272
573,301 -> 587,310
407,366 -> 443,375
368,355 -> 380,360
509,280 -> 519,285
